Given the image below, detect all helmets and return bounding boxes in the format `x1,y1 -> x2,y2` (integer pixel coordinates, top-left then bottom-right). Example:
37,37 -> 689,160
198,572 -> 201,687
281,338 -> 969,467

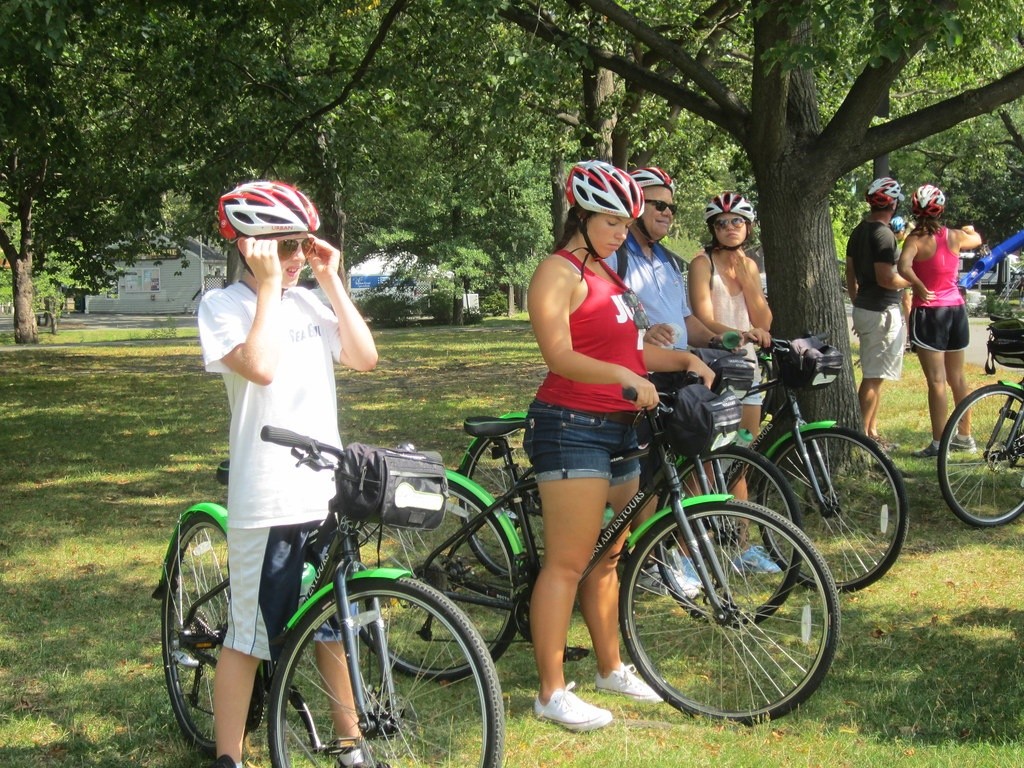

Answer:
705,192 -> 755,221
566,160 -> 646,218
912,185 -> 946,219
631,166 -> 677,198
865,177 -> 902,209
217,180 -> 321,240
889,215 -> 905,233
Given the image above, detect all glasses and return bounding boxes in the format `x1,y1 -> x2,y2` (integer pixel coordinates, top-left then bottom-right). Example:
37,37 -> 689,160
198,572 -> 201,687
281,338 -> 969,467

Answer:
622,289 -> 649,331
712,218 -> 749,228
646,199 -> 680,215
276,237 -> 317,257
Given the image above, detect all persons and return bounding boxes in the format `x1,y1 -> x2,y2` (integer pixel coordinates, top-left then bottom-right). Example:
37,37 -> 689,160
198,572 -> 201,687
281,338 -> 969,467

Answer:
890,217 -> 916,333
197,182 -> 378,768
526,159 -> 716,730
605,167 -> 744,598
898,185 -> 981,456
678,192 -> 781,585
846,177 -> 912,452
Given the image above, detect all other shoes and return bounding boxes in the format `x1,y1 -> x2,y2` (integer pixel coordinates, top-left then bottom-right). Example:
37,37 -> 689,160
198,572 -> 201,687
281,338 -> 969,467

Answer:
208,753 -> 238,768
333,741 -> 391,768
905,334 -> 912,350
868,436 -> 899,453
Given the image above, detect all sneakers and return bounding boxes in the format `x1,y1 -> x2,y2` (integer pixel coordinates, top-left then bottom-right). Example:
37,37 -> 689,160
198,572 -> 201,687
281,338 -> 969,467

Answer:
634,562 -> 701,599
534,681 -> 613,733
911,444 -> 950,461
732,546 -> 783,574
673,557 -> 702,587
595,663 -> 667,704
949,435 -> 977,454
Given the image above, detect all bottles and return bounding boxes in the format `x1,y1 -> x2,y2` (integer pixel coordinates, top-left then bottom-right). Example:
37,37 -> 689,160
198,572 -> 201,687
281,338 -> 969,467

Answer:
601,502 -> 615,536
298,562 -> 317,610
754,414 -> 773,441
720,428 -> 753,473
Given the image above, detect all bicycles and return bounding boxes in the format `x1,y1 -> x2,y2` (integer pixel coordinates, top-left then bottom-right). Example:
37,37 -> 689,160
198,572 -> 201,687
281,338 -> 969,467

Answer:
937,271 -> 1024,528
455,331 -> 805,630
679,336 -> 911,596
336,362 -> 842,728
150,425 -> 506,768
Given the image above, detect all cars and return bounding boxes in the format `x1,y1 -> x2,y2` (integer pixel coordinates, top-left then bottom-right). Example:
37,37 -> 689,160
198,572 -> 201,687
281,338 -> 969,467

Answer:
957,252 -> 1023,288
742,244 -> 768,298
